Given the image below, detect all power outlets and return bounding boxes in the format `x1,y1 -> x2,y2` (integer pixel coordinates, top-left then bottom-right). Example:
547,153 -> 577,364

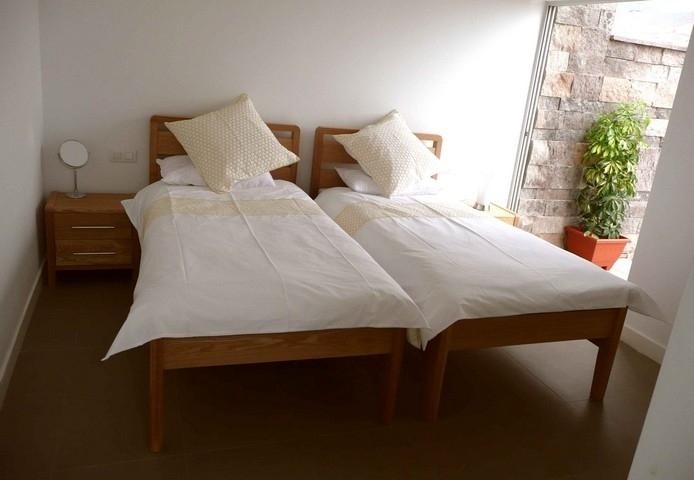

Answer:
112,151 -> 123,162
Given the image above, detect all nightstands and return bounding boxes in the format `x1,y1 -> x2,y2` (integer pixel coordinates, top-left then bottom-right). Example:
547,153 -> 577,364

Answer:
486,202 -> 518,226
44,191 -> 139,289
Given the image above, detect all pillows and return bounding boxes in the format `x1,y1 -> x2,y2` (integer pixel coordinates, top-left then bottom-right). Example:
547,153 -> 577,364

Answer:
336,167 -> 445,193
154,156 -> 275,186
333,110 -> 447,196
164,94 -> 301,193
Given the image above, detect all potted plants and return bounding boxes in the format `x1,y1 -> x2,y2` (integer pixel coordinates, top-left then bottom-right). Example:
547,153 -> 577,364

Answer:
565,97 -> 651,271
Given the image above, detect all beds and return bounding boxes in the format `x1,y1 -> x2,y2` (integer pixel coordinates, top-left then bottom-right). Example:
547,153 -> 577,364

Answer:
309,127 -> 672,423
99,116 -> 432,453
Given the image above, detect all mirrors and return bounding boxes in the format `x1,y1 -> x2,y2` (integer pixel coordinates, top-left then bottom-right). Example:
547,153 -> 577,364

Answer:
58,138 -> 91,199
